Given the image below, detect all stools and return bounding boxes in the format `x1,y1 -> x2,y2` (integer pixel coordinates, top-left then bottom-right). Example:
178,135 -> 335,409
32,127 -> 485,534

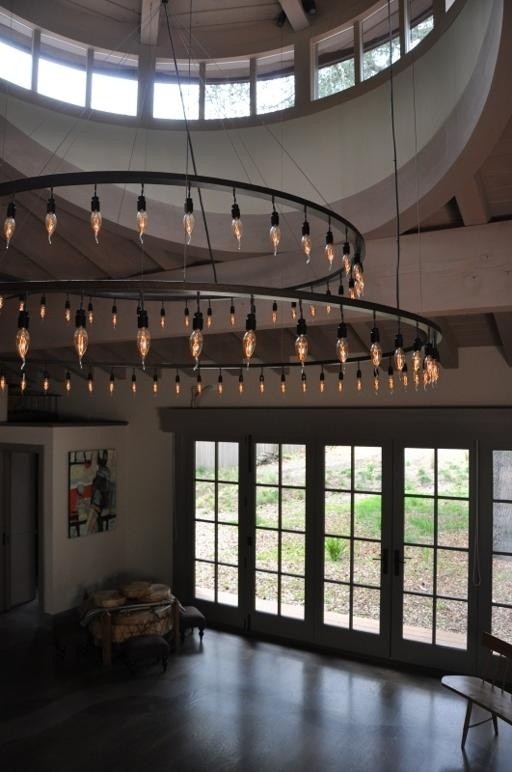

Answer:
130,637 -> 171,671
178,603 -> 206,641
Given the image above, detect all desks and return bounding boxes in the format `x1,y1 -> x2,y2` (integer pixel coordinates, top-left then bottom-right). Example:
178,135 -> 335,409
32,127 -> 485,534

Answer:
81,578 -> 178,670
433,670 -> 512,753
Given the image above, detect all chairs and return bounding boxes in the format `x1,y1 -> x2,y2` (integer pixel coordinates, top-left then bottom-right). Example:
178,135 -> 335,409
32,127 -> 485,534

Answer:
478,632 -> 512,688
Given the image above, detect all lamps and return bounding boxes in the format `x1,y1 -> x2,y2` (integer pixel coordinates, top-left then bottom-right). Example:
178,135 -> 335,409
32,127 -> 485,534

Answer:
0,1 -> 453,396
